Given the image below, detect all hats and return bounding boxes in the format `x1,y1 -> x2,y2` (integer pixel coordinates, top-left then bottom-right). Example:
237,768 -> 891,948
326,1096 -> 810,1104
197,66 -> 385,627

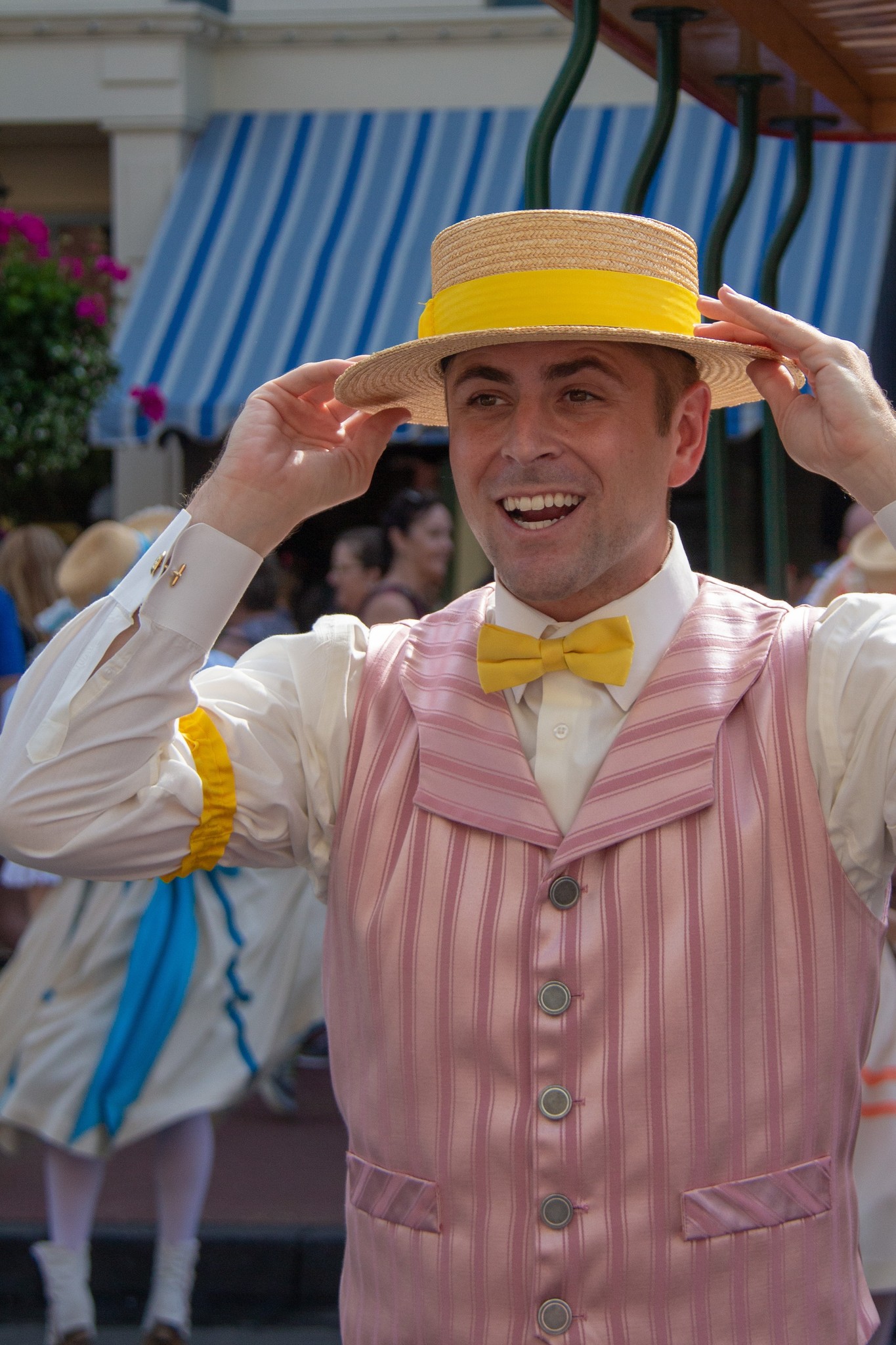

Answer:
31,501 -> 180,635
334,210 -> 809,430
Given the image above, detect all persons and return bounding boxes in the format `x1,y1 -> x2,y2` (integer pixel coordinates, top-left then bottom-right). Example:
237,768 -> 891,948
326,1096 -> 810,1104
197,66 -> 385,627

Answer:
2,494 -> 452,1116
796,501 -> 896,1300
1,505 -> 327,1345
0,208 -> 896,1345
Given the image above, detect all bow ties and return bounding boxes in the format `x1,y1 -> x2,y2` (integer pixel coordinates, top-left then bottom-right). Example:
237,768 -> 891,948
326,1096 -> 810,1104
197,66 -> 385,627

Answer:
480,617 -> 638,695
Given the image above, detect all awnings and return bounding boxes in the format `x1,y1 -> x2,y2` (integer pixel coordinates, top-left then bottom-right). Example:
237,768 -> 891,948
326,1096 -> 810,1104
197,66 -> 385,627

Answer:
89,109 -> 896,444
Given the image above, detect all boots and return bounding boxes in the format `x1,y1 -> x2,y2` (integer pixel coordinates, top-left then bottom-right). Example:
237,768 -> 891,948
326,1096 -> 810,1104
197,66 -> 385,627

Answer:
142,1236 -> 203,1345
30,1237 -> 94,1345
